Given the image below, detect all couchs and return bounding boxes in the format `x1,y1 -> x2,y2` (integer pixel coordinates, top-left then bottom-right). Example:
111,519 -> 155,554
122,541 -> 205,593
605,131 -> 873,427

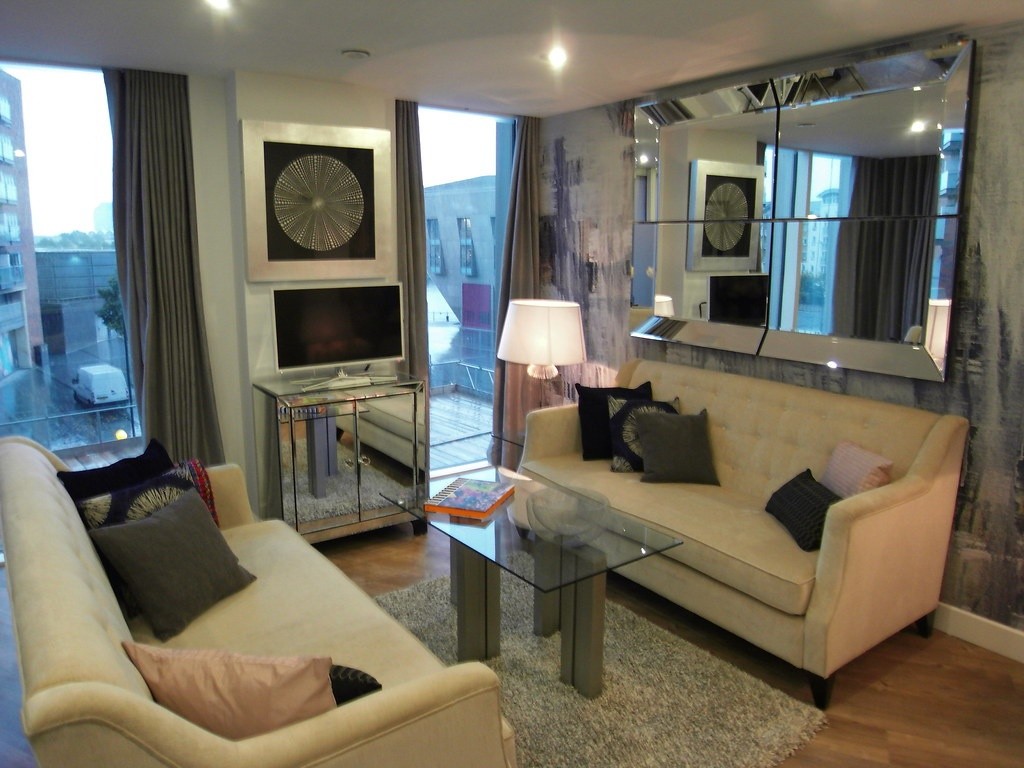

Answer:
512,357 -> 969,710
0,434 -> 520,767
336,393 -> 425,472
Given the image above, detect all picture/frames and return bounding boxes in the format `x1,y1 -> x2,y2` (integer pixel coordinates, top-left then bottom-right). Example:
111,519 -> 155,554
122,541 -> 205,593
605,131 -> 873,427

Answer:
240,118 -> 391,280
689,158 -> 760,271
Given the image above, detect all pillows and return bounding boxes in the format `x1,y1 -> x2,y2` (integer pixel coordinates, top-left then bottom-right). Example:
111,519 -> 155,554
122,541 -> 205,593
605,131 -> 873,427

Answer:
325,666 -> 384,700
634,409 -> 722,486
575,383 -> 651,461
77,466 -> 202,618
764,467 -> 848,553
820,441 -> 894,503
607,397 -> 679,473
53,438 -> 172,501
121,640 -> 336,742
84,487 -> 257,642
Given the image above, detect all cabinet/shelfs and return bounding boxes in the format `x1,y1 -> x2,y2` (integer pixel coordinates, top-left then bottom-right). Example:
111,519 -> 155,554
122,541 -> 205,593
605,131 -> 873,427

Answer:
252,380 -> 430,555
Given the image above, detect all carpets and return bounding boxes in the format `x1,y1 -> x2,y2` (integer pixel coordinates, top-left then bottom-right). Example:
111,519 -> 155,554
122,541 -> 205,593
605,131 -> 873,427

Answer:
371,550 -> 826,766
279,436 -> 408,520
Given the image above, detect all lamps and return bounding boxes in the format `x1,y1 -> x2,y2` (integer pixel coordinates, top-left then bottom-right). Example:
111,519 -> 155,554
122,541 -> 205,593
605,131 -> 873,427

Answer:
498,298 -> 588,411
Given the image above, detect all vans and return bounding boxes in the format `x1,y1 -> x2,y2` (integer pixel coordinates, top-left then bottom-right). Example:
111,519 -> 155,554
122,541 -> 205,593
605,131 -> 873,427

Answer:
72,363 -> 130,416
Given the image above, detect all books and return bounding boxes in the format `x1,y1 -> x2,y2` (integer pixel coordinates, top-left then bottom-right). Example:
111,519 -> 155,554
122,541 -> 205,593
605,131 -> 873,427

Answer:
424,477 -> 515,521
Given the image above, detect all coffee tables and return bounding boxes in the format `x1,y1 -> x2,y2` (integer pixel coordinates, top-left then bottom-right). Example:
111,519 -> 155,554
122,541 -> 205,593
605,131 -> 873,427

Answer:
279,404 -> 370,496
373,466 -> 684,694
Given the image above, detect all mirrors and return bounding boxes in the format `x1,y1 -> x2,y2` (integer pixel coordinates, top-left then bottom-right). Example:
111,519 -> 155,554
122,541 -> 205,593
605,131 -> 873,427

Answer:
627,40 -> 975,384
